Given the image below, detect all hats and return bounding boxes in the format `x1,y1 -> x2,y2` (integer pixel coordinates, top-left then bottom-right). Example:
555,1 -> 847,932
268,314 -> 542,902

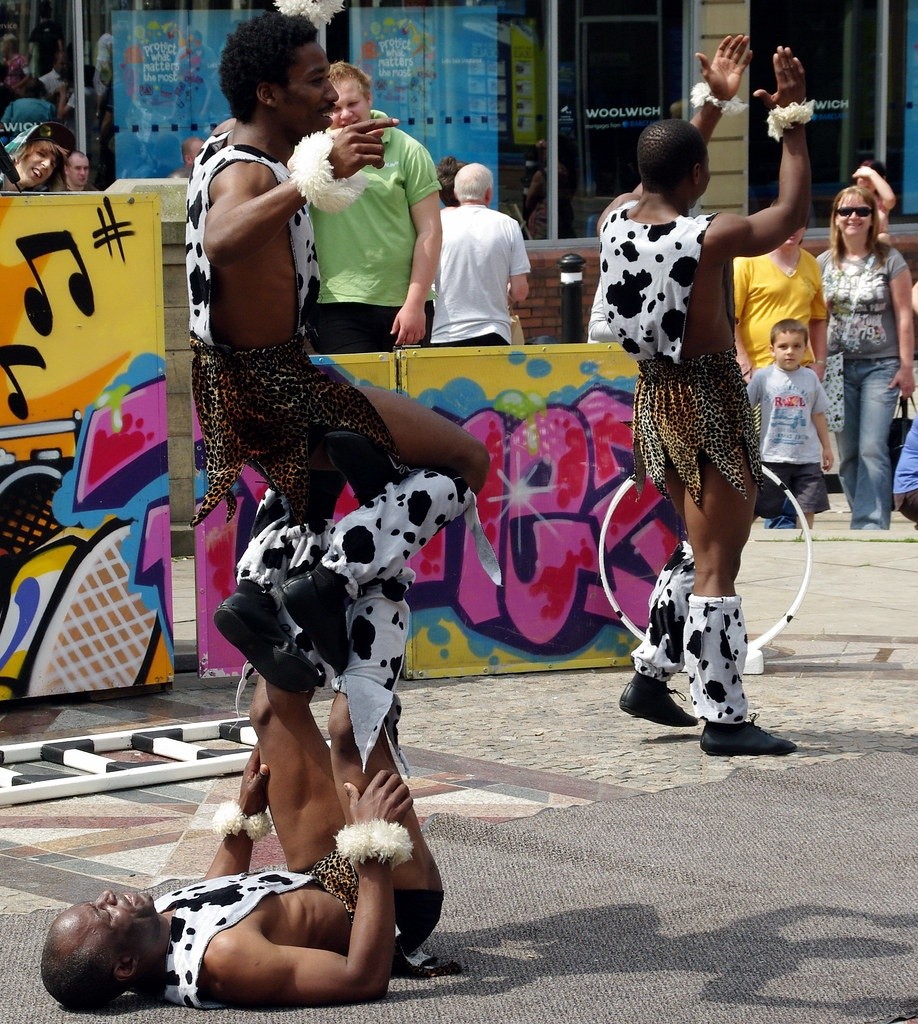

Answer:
11,122 -> 77,167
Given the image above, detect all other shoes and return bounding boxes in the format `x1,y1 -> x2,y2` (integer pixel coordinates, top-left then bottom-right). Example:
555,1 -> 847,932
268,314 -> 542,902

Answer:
617,683 -> 699,728
213,593 -> 320,693
280,569 -> 349,673
322,431 -> 393,492
700,713 -> 797,756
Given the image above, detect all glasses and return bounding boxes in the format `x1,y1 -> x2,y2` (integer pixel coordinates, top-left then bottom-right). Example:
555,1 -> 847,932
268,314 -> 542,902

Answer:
835,206 -> 872,217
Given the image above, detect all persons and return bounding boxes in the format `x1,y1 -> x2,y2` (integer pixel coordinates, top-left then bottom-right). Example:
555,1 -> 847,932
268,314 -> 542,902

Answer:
0,0 -> 112,194
426,154 -> 532,347
286,58 -> 442,355
743,318 -> 835,529
168,136 -> 206,178
590,160 -> 917,529
600,33 -> 813,755
525,133 -> 579,240
40,431 -> 443,1012
181,0 -> 490,696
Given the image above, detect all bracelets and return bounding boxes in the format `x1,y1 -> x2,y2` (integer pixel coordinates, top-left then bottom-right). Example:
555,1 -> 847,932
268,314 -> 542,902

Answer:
688,82 -> 747,117
274,0 -> 345,28
768,99 -> 816,141
334,818 -> 413,869
211,800 -> 271,842
288,131 -> 368,213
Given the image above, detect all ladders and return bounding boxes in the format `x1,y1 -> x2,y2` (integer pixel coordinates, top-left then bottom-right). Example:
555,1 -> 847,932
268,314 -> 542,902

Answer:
0,716 -> 257,808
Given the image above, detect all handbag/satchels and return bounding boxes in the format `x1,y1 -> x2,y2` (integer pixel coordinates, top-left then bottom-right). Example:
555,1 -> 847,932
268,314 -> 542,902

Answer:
889,396 -> 917,455
